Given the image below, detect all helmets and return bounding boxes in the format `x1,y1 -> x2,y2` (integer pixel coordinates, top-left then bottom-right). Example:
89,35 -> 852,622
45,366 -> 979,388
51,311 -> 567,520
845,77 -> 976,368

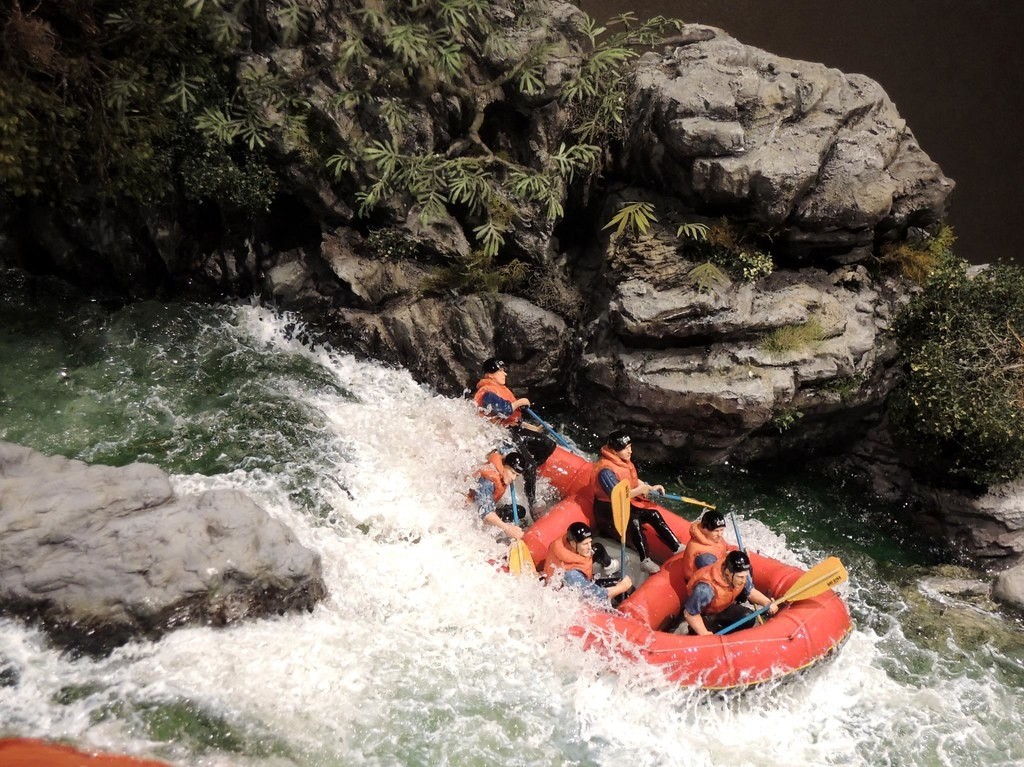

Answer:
505,451 -> 526,475
724,550 -> 750,589
701,510 -> 726,531
482,357 -> 508,374
607,431 -> 633,451
566,521 -> 594,554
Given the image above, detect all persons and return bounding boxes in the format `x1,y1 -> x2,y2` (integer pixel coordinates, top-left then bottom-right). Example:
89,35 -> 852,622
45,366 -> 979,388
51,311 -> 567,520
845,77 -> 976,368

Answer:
473,357 -> 556,520
673,510 -> 779,636
590,430 -> 687,573
458,450 -> 525,540
543,522 -> 632,613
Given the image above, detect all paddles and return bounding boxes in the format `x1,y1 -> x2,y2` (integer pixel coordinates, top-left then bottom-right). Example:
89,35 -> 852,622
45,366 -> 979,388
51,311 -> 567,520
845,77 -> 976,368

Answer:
525,406 -> 583,455
508,480 -> 537,576
646,489 -> 715,509
729,507 -> 765,627
610,478 -> 631,600
713,556 -> 847,635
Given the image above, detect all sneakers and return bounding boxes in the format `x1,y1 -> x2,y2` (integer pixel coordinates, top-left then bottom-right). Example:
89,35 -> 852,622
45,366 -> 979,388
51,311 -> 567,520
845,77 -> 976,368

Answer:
528,499 -> 547,522
640,557 -> 660,574
605,555 -> 629,576
672,543 -> 686,555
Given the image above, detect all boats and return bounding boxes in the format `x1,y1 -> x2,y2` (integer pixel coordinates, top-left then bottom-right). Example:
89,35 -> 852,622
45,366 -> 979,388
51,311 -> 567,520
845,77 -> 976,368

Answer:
455,419 -> 852,697
